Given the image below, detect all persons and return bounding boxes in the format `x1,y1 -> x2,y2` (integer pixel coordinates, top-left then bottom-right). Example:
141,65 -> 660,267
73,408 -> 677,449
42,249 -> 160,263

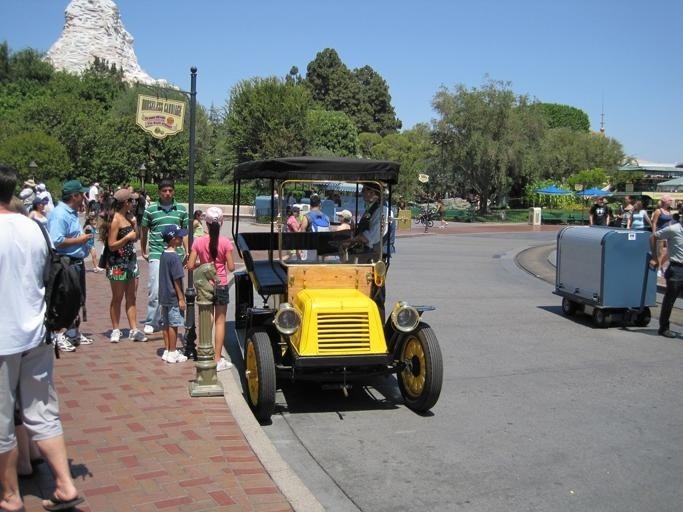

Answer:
7,196 -> 45,477
20,179 -> 236,372
396,195 -> 407,211
274,188 -> 278,199
327,182 -> 396,326
285,188 -> 354,263
0,162 -> 84,512
435,197 -> 449,230
589,193 -> 683,338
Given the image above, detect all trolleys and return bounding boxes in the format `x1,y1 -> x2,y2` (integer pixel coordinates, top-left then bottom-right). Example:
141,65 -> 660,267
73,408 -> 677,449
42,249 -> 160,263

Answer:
544,250 -> 657,327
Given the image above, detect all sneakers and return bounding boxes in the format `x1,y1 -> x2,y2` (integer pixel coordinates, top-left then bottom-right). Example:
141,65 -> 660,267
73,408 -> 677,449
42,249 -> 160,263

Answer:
51,334 -> 76,351
67,334 -> 94,344
128,330 -> 148,341
217,359 -> 233,372
663,330 -> 676,337
161,350 -> 188,363
144,325 -> 155,335
110,329 -> 120,342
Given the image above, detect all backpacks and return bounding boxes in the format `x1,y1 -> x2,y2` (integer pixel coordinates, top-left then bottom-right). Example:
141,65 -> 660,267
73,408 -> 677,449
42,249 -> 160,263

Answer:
32,218 -> 81,331
309,212 -> 330,232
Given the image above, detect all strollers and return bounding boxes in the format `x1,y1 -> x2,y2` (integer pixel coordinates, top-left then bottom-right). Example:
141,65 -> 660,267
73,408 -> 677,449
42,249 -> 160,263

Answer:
414,208 -> 438,228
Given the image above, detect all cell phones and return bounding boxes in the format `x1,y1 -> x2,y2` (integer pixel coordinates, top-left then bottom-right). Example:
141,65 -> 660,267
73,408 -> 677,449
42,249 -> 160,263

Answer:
86,229 -> 91,240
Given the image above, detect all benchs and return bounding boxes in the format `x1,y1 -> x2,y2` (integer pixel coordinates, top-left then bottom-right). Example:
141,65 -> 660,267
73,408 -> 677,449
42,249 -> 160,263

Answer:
410,206 -> 423,217
539,211 -> 590,226
443,207 -> 465,222
235,232 -> 359,310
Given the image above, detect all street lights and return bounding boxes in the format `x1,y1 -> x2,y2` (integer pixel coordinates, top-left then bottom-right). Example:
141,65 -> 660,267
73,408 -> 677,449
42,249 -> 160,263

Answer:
136,163 -> 148,191
28,160 -> 39,185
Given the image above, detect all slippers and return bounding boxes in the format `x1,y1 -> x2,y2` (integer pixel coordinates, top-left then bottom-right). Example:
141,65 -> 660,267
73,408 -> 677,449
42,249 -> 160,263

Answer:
44,492 -> 84,511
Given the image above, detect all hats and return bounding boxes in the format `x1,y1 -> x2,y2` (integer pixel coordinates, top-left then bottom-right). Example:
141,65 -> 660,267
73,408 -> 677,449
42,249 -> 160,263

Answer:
335,210 -> 353,222
33,198 -> 48,205
62,181 -> 89,196
114,189 -> 140,201
24,180 -> 36,187
205,207 -> 224,225
162,225 -> 188,241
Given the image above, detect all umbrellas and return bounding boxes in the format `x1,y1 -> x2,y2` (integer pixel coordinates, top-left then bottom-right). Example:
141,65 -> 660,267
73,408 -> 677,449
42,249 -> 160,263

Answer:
578,187 -> 608,199
534,186 -> 569,209
